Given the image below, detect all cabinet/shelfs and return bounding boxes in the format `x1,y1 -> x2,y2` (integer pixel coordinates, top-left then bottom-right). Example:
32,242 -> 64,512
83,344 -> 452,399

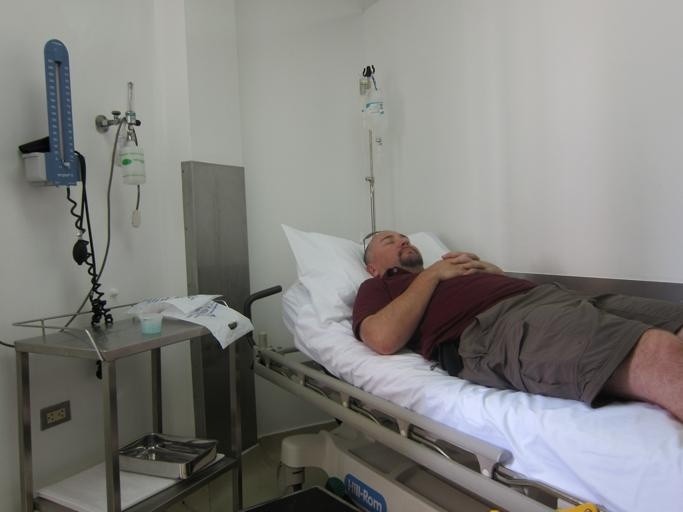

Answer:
11,299 -> 243,511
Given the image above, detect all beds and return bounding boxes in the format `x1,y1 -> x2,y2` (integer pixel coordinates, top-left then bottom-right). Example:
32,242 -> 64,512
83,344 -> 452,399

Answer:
252,249 -> 680,510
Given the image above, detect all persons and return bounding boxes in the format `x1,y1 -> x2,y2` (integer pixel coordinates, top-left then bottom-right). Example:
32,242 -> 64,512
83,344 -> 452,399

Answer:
352,231 -> 683,421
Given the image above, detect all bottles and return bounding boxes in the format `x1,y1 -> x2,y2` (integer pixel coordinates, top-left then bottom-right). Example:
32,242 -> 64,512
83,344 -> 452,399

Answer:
365,90 -> 384,143
121,139 -> 146,185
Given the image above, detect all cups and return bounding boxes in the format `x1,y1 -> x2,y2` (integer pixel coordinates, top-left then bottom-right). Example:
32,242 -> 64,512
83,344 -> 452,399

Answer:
141,312 -> 161,333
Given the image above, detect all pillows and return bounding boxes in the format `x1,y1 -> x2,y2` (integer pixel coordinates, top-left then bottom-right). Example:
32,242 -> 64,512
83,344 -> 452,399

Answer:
282,222 -> 456,321
